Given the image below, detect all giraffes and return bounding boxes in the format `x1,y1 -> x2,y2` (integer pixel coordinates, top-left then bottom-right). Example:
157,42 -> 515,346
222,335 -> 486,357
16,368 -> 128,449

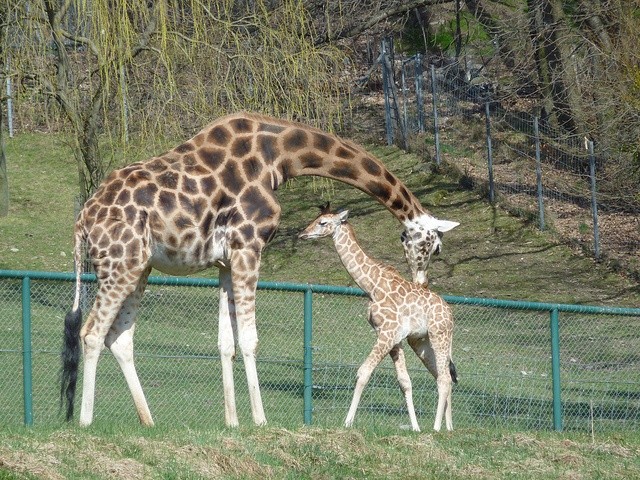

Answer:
297,208 -> 458,432
57,110 -> 461,429
